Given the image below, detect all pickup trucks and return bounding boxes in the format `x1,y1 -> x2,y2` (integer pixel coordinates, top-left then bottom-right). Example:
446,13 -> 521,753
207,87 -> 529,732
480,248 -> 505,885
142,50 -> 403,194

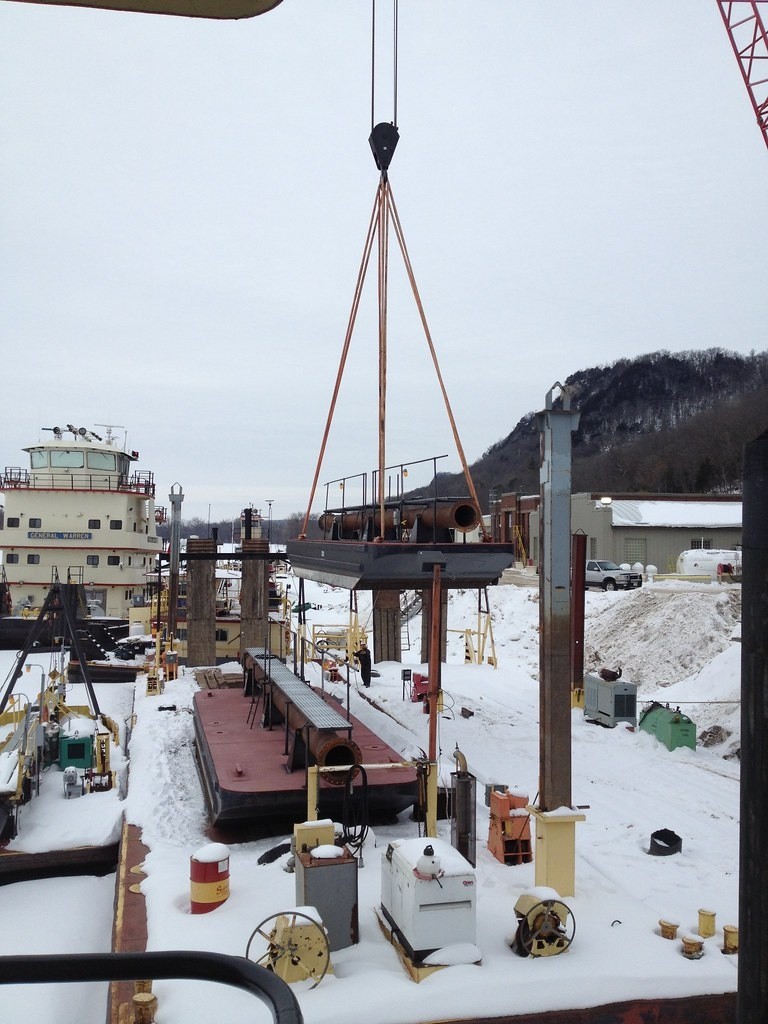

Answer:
570,560 -> 643,592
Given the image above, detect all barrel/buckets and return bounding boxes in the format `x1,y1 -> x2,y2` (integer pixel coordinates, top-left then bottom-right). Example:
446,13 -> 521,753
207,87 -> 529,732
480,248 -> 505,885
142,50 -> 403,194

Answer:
190,855 -> 230,914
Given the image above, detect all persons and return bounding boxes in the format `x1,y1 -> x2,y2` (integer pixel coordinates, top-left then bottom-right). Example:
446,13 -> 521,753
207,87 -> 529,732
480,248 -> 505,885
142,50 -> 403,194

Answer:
351,640 -> 371,688
392,506 -> 400,540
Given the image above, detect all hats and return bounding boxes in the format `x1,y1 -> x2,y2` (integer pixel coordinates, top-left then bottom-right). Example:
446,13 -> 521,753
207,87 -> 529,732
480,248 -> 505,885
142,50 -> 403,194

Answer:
360,641 -> 366,645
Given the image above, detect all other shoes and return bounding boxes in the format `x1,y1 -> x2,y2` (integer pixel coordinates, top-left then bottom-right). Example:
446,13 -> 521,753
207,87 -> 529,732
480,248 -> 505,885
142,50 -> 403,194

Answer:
366,684 -> 369,688
